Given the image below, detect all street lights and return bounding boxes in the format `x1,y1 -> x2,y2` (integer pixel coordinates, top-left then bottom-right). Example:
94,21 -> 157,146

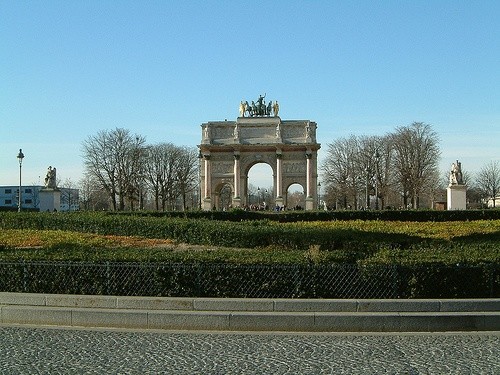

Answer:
364,167 -> 371,210
15,149 -> 26,212
318,182 -> 322,210
373,149 -> 382,209
264,189 -> 267,204
257,186 -> 261,206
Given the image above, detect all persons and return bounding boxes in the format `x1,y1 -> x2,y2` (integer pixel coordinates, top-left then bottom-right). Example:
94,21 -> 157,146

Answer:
238,92 -> 280,118
44,165 -> 57,190
188,200 -> 414,213
449,159 -> 466,186
